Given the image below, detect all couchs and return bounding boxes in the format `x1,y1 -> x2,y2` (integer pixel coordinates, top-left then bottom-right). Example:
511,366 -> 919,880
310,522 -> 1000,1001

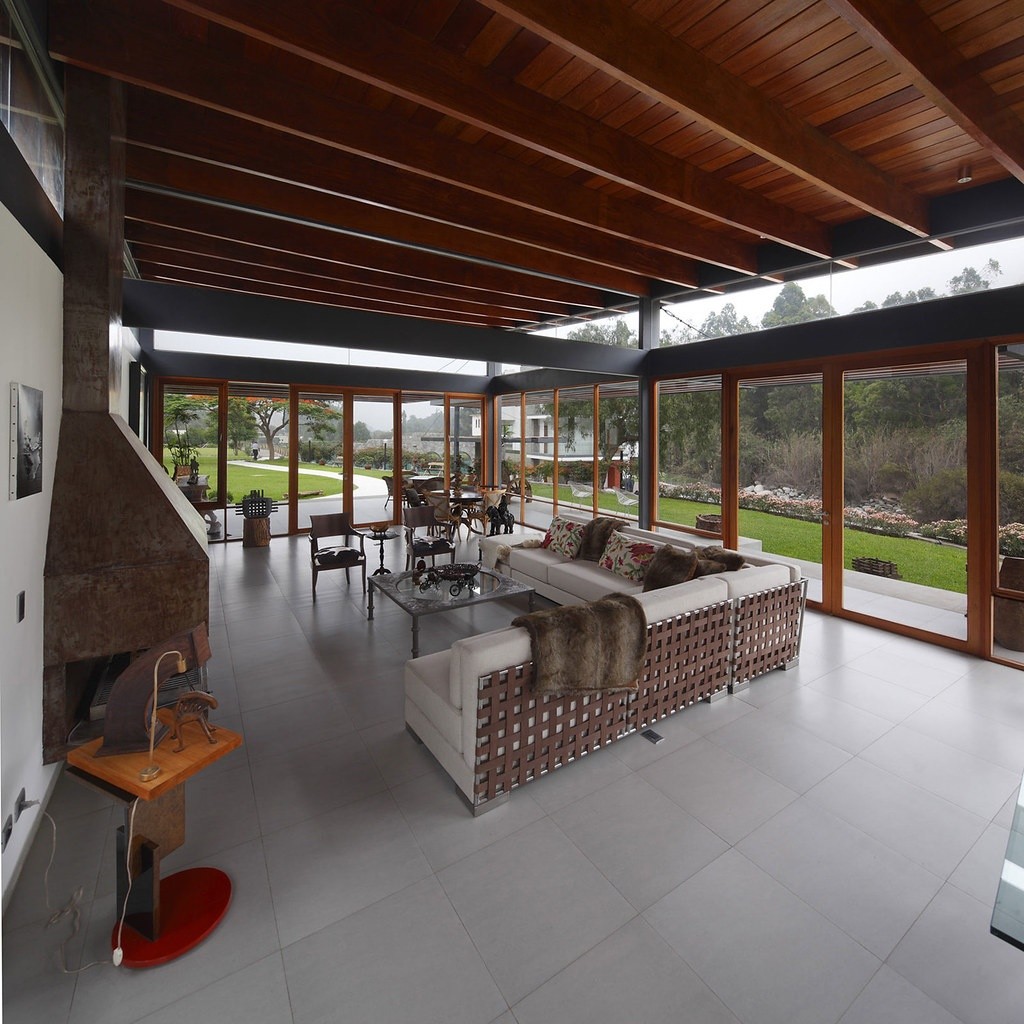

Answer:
404,514 -> 809,818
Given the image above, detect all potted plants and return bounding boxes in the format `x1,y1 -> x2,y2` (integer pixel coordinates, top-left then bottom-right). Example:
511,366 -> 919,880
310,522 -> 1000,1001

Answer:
164,405 -> 201,475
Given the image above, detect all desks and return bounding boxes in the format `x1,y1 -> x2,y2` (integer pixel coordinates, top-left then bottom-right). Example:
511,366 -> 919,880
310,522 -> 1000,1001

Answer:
434,490 -> 482,539
63,708 -> 243,968
175,474 -> 222,534
367,561 -> 535,660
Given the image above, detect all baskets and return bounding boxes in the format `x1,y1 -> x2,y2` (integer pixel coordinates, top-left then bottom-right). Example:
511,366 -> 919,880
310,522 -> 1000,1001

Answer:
695,513 -> 722,533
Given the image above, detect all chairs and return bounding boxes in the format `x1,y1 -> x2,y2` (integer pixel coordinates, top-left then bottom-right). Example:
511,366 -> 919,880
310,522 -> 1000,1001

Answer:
308,512 -> 366,601
382,471 -> 507,571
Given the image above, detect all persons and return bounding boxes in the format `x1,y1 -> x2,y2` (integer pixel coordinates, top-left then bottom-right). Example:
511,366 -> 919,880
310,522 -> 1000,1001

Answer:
252,442 -> 260,459
497,495 -> 507,517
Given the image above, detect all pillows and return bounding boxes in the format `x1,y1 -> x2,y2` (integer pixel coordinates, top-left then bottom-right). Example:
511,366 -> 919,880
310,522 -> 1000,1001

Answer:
642,544 -> 697,593
694,558 -> 727,579
598,530 -> 660,583
698,545 -> 744,572
541,515 -> 587,560
